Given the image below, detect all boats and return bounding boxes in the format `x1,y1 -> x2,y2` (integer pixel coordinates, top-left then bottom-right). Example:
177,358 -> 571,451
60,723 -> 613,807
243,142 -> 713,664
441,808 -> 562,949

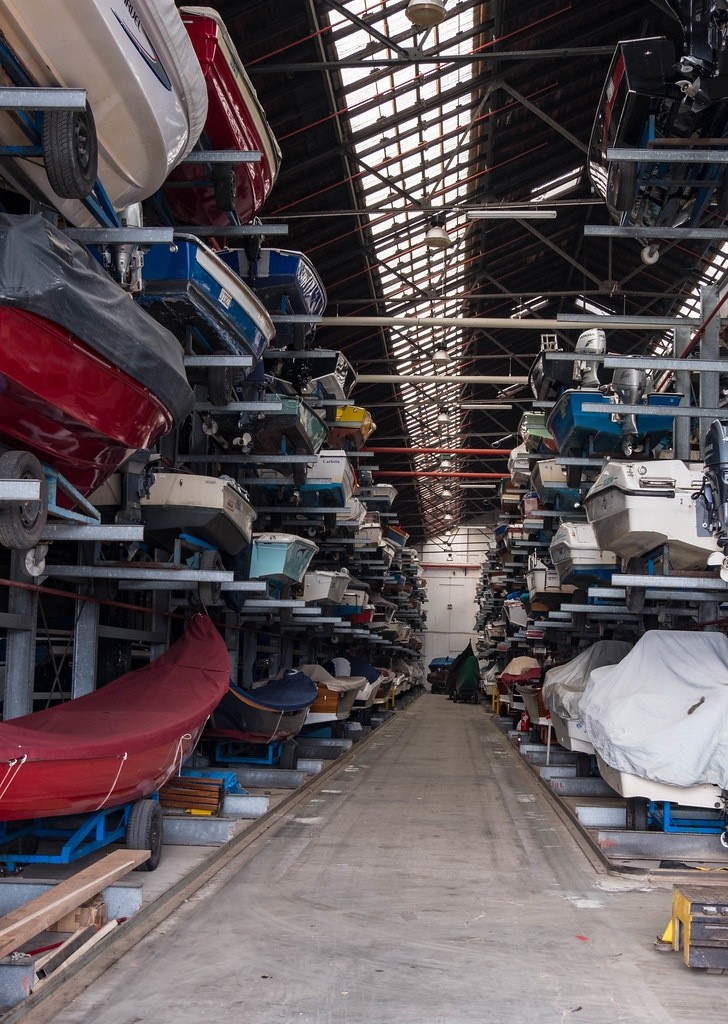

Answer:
585,34 -> 727,258
84,235 -> 278,390
293,664 -> 368,728
0,213 -> 199,519
582,454 -> 720,572
201,373 -> 328,475
214,248 -> 328,348
541,641 -> 634,755
0,0 -> 210,227
153,5 -> 286,235
251,528 -> 321,589
199,669 -> 320,744
242,350 -> 425,710
582,631 -> 727,812
544,386 -> 683,465
528,347 -> 615,411
79,469 -> 260,557
0,614 -> 232,821
468,411 -> 619,748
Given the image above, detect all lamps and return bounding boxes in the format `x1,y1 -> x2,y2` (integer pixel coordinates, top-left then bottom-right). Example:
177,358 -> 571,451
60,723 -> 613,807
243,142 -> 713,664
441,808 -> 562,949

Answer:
441,486 -> 453,496
445,543 -> 453,552
444,527 -> 452,535
444,511 -> 452,520
447,554 -> 453,561
440,460 -> 452,468
457,525 -> 486,529
467,212 -> 556,219
405,0 -> 447,26
423,215 -> 451,247
432,346 -> 452,363
460,404 -> 512,410
437,407 -> 450,423
460,485 -> 496,489
